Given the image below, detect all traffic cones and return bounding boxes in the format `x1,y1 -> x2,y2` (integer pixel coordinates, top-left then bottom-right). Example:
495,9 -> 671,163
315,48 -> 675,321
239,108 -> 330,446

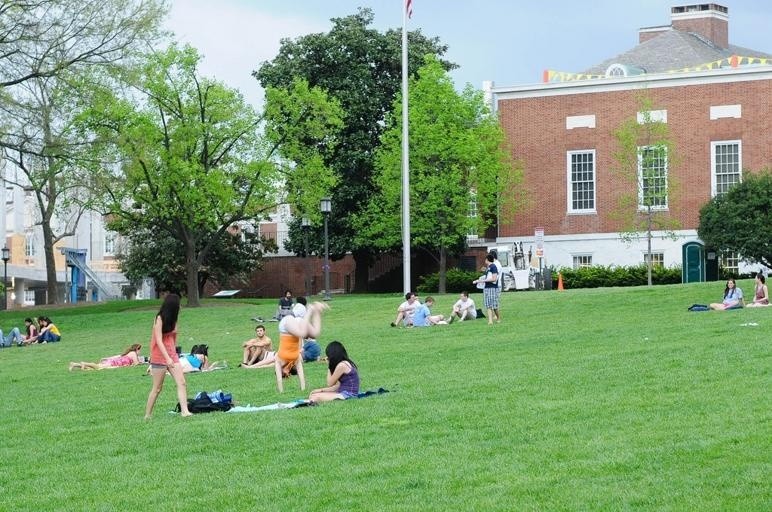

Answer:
557,273 -> 564,291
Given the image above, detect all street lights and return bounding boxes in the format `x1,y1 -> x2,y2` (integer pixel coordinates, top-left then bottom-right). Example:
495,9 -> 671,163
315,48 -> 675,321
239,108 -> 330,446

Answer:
1,242 -> 10,310
301,193 -> 331,301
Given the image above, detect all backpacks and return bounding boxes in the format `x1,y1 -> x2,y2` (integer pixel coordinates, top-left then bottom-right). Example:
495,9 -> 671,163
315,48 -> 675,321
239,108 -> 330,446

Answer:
191,344 -> 208,355
175,389 -> 233,414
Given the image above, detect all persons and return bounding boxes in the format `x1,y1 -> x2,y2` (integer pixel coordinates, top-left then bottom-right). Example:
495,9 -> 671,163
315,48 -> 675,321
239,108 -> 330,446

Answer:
2,316 -> 61,347
390,251 -> 503,325
309,341 -> 358,402
709,278 -> 744,310
745,274 -> 772,307
145,294 -> 193,416
275,291 -> 307,320
240,325 -> 321,368
148,343 -> 208,375
274,301 -> 327,392
69,343 -> 142,371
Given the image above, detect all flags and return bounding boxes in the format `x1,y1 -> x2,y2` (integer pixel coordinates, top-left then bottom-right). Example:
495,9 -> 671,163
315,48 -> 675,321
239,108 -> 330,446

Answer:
405,0 -> 412,19
